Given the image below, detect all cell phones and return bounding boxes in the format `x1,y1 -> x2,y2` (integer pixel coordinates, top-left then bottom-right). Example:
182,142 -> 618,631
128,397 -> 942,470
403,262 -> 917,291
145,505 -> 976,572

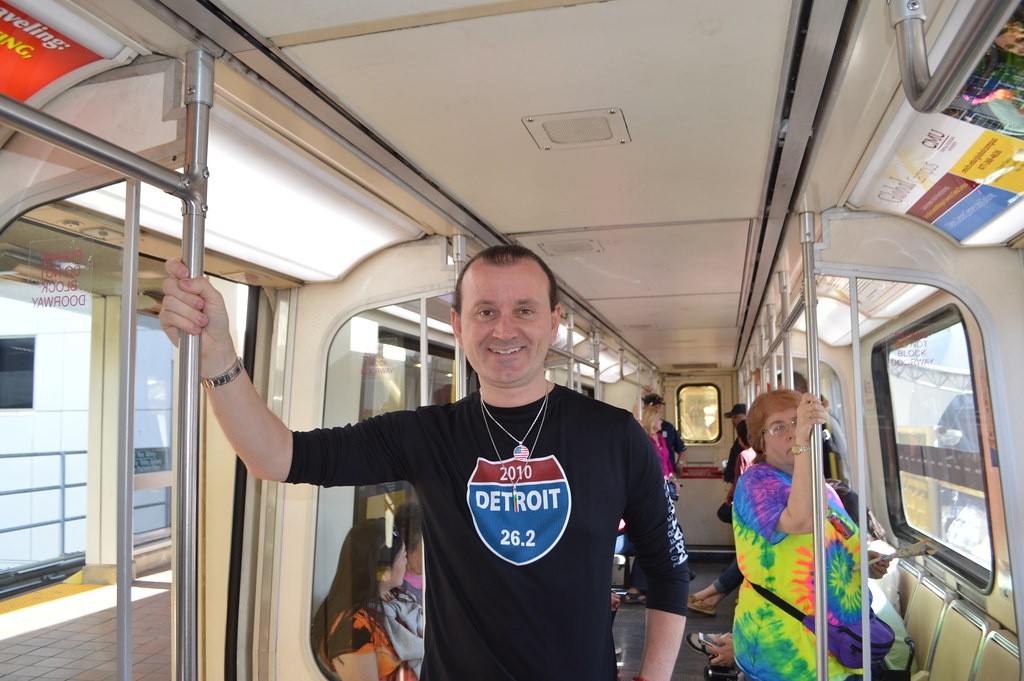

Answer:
698,632 -> 725,648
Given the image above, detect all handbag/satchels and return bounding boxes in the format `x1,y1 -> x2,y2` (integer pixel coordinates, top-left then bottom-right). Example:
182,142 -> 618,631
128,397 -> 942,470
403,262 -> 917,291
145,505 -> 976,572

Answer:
749,581 -> 895,668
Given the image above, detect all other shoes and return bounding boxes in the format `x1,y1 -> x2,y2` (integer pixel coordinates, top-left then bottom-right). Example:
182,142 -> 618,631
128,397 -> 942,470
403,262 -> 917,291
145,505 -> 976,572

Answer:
686,633 -> 718,660
686,594 -> 696,603
686,601 -> 716,615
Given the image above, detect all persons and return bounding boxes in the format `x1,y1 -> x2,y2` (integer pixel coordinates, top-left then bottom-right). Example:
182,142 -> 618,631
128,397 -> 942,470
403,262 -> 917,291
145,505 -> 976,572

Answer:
632,384 -> 688,497
312,516 -> 417,681
159,242 -> 695,681
381,501 -> 427,681
732,388 -> 892,681
686,370 -> 873,616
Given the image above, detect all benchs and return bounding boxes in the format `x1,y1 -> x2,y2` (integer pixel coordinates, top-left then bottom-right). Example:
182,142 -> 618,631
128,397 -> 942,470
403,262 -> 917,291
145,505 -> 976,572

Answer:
891,558 -> 1022,681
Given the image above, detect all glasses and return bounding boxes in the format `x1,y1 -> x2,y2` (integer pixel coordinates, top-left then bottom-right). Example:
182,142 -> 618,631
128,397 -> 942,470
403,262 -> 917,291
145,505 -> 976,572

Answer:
379,523 -> 399,550
760,420 -> 798,437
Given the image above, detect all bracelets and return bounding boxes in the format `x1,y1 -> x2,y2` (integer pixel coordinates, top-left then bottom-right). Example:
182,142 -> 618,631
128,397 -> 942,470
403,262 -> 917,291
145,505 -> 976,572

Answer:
790,444 -> 811,454
677,460 -> 684,466
200,357 -> 245,389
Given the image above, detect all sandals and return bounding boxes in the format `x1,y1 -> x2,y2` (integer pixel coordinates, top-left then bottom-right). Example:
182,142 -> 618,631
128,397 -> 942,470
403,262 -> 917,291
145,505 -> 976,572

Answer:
624,592 -> 646,603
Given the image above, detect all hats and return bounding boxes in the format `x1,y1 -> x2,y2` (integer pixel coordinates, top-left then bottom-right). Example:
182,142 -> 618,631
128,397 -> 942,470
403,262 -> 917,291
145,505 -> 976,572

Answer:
642,393 -> 665,407
723,404 -> 746,418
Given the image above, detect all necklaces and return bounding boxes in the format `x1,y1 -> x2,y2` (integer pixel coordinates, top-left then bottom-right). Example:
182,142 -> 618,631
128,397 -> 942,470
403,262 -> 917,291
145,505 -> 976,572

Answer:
479,381 -> 549,512
